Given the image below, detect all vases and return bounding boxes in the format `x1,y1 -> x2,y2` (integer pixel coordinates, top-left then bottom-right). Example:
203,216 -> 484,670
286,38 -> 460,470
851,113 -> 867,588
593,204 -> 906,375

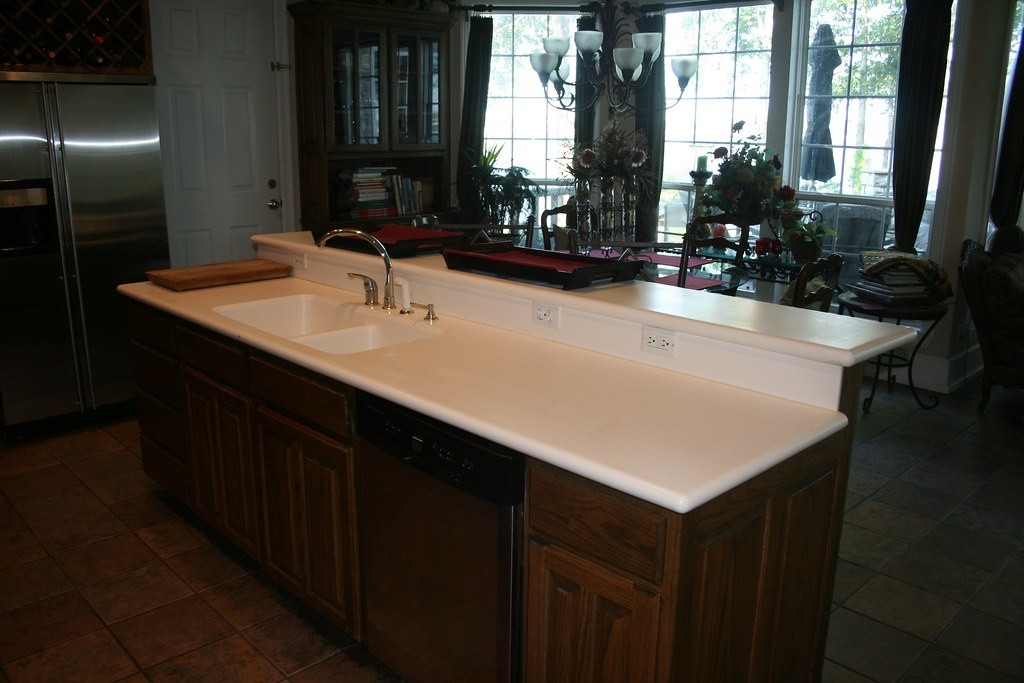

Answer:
734,209 -> 767,226
791,237 -> 822,263
575,175 -> 637,253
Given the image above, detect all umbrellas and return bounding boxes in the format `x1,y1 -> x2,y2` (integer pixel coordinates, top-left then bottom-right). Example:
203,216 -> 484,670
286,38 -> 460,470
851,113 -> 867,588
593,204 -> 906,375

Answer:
800,24 -> 842,190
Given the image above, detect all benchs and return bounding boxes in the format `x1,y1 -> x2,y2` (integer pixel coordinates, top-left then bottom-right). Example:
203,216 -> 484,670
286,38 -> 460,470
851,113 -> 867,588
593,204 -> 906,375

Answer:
958,224 -> 1024,415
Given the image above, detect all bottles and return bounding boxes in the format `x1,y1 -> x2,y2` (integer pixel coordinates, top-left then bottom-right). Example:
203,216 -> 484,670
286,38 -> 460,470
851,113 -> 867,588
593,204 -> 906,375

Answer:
11,17 -> 112,65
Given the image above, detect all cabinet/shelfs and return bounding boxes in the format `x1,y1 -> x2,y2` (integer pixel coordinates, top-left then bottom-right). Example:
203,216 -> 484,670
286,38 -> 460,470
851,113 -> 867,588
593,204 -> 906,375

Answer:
131,301 -> 362,645
0,0 -> 153,75
286,0 -> 461,251
522,456 -> 838,683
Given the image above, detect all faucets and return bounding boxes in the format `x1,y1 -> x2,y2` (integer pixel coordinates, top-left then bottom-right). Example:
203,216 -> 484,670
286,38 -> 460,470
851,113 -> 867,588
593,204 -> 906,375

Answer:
317,227 -> 397,309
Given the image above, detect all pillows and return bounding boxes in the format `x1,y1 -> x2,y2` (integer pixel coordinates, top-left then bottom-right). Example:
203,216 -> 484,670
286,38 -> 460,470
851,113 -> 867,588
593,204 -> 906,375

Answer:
777,278 -> 827,311
553,223 -> 602,249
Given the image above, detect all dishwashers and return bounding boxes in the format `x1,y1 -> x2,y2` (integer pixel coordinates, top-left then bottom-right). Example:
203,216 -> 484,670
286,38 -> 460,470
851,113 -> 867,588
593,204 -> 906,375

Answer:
356,386 -> 524,683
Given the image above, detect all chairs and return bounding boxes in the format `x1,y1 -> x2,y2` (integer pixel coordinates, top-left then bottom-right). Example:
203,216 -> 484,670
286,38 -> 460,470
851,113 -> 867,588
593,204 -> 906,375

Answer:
683,215 -> 751,295
541,204 -> 598,251
793,253 -> 843,312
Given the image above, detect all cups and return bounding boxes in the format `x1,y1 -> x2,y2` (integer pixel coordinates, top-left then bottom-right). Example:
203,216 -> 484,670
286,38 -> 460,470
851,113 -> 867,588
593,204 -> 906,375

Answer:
756,237 -> 782,254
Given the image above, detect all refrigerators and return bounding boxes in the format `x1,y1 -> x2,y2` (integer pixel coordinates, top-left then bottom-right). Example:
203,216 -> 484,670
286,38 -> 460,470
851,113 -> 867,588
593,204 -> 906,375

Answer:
0,71 -> 171,428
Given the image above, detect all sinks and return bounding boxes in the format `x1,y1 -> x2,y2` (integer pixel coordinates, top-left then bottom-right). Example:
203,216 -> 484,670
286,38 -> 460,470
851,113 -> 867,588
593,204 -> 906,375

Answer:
209,295 -> 368,338
294,322 -> 446,355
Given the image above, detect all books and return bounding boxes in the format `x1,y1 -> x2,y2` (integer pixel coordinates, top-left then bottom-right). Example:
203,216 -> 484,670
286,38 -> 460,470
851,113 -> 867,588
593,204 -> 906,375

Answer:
348,167 -> 422,218
855,265 -> 925,294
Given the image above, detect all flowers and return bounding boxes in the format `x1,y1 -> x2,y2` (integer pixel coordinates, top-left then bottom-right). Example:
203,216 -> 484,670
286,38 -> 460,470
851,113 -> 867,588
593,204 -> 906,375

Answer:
550,115 -> 660,238
702,133 -> 841,253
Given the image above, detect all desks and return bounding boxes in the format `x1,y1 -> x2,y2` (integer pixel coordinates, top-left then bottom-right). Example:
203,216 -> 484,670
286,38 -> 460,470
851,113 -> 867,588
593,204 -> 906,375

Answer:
557,248 -> 749,297
657,246 -> 814,284
838,292 -> 947,412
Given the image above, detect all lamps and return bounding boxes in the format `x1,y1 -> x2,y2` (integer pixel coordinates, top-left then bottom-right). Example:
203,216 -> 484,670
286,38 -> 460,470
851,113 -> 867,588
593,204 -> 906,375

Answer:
529,0 -> 699,113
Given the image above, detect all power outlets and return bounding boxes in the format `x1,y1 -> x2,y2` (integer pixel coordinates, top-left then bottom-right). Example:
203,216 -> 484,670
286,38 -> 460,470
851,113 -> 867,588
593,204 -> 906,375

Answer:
640,325 -> 679,360
532,301 -> 561,330
